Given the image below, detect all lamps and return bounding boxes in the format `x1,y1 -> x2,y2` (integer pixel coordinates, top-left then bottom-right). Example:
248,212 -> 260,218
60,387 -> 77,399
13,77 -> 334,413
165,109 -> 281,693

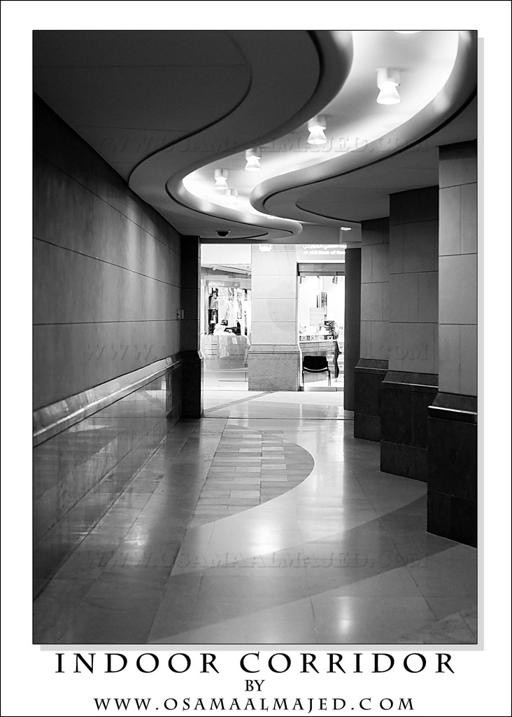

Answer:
209,68 -> 404,191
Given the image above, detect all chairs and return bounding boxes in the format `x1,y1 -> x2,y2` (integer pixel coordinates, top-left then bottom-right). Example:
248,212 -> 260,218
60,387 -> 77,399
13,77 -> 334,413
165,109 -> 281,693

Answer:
301,354 -> 331,386
